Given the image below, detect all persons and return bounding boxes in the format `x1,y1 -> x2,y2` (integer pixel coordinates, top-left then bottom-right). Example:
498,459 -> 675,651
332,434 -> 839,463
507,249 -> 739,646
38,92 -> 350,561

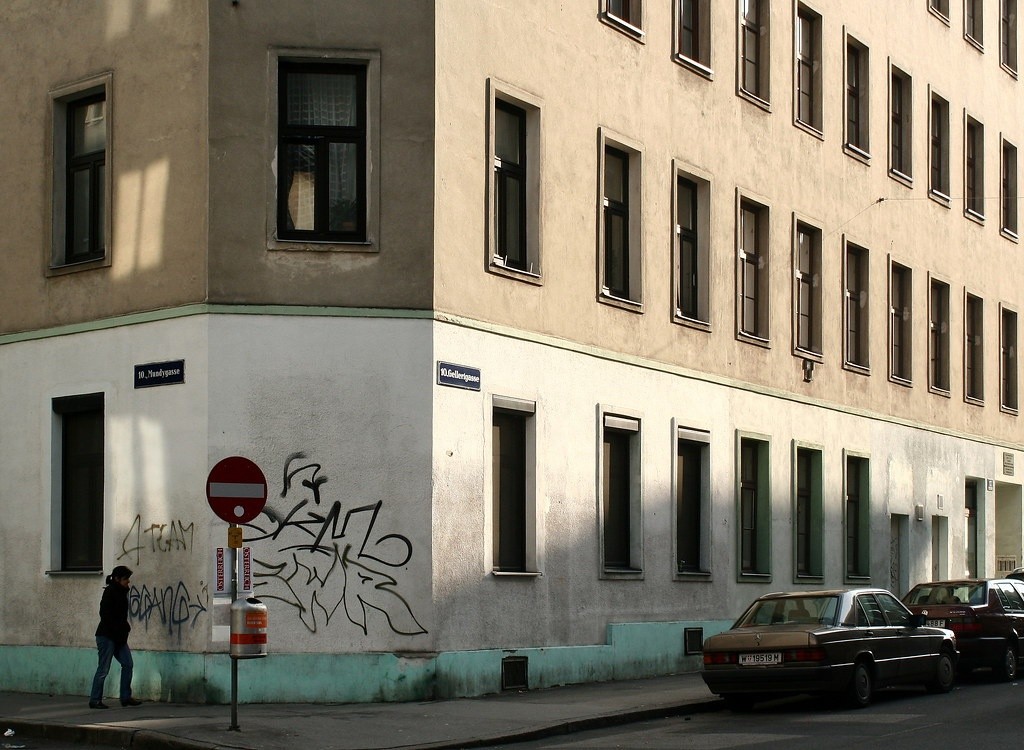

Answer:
89,565 -> 144,709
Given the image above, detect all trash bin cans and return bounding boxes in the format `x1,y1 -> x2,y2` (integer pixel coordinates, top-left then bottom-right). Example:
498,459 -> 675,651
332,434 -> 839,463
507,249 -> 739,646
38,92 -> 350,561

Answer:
230,597 -> 269,660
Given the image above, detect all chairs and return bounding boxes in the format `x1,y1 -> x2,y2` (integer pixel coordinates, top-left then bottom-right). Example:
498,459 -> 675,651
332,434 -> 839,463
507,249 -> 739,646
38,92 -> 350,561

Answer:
787,609 -> 810,624
916,595 -> 938,605
942,596 -> 961,605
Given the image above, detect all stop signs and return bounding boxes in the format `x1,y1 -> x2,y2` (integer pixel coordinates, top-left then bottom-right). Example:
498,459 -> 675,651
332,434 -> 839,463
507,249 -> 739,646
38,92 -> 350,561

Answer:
207,457 -> 268,524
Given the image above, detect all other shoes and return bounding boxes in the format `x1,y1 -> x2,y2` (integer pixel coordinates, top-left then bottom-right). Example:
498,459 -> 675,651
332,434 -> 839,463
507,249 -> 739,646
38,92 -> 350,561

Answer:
89,702 -> 109,709
121,698 -> 142,707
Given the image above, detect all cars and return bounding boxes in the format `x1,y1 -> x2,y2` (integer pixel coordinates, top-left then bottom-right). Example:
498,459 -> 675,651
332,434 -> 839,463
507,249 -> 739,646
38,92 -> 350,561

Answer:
900,577 -> 1024,680
701,586 -> 956,708
